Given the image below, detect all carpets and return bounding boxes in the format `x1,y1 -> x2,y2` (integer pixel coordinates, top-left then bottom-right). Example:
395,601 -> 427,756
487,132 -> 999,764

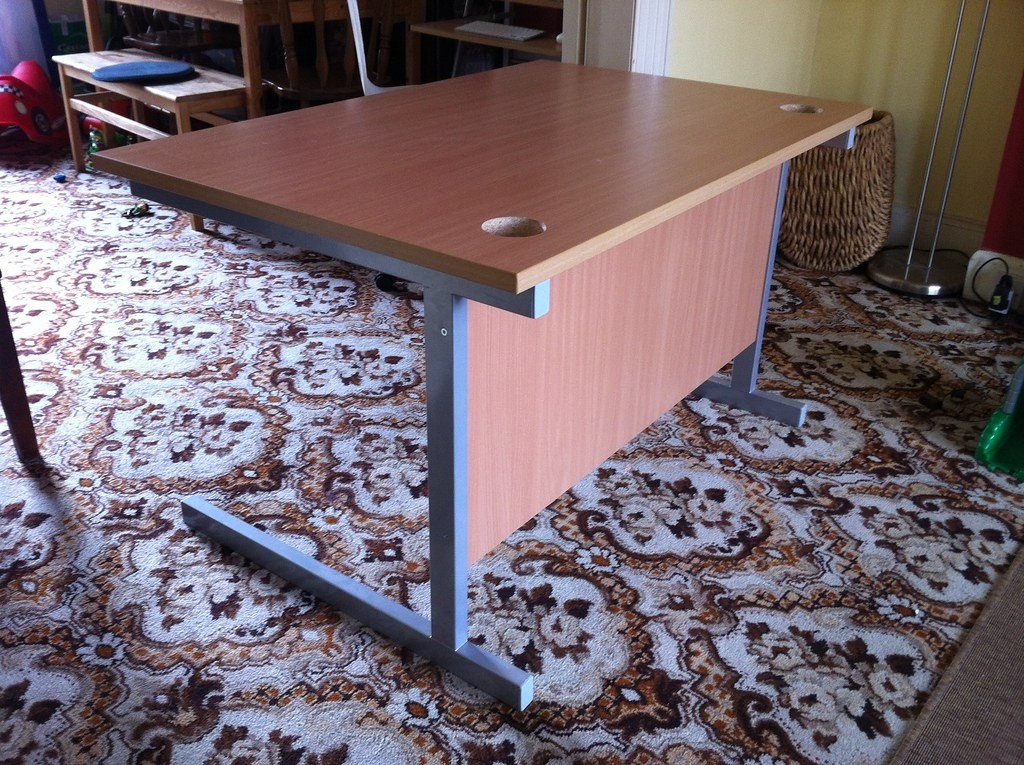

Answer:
0,135 -> 1024,765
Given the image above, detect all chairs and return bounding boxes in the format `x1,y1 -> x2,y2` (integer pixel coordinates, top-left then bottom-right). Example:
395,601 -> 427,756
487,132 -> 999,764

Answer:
408,0 -> 563,80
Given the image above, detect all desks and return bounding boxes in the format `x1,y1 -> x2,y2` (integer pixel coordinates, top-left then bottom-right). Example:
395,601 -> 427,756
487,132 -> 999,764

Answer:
83,0 -> 426,153
89,59 -> 876,712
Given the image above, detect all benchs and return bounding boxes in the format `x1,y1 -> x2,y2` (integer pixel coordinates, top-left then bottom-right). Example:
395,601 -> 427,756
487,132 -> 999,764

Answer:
52,48 -> 268,230
